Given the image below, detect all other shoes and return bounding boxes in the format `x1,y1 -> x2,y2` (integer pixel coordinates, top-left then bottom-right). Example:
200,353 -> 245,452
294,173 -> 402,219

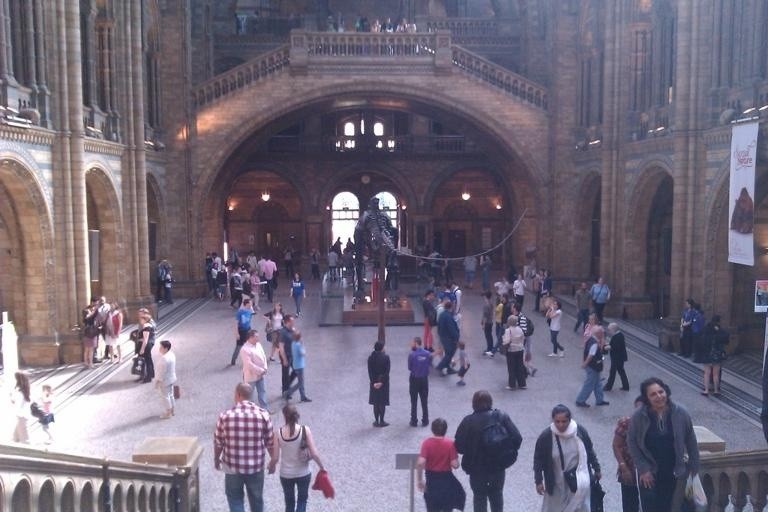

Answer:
560,350 -> 565,356
576,400 -> 590,408
457,381 -> 466,384
597,400 -> 609,405
530,367 -> 537,376
700,391 -> 708,396
505,386 -> 516,389
373,420 -> 389,426
547,353 -> 557,357
484,351 -> 495,358
712,392 -> 719,397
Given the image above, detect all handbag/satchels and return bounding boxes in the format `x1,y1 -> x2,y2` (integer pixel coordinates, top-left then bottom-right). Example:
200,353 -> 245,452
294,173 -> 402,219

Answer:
131,358 -> 145,376
563,465 -> 598,493
297,446 -> 312,463
174,386 -> 179,399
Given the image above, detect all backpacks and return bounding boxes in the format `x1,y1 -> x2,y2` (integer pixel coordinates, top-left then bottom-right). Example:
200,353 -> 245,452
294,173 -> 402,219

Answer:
518,316 -> 533,336
482,410 -> 516,469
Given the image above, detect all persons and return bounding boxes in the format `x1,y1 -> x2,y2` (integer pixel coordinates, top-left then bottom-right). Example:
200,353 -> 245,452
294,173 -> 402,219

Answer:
11,369 -> 53,413
626,377 -> 701,512
452,388 -> 524,512
369,246 -> 731,423
81,295 -> 123,369
416,417 -> 464,512
324,10 -> 419,56
531,404 -> 602,512
608,394 -> 644,512
132,238 -> 357,512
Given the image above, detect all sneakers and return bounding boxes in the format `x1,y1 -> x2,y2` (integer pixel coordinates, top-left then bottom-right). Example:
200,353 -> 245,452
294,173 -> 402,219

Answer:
229,357 -> 313,409
159,411 -> 175,419
